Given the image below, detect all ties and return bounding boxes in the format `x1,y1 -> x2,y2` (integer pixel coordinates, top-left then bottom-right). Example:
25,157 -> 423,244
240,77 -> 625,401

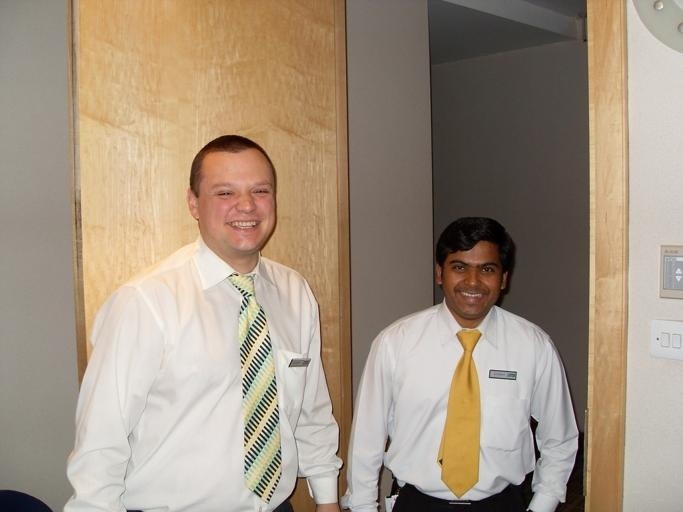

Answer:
228,272 -> 283,504
436,329 -> 482,499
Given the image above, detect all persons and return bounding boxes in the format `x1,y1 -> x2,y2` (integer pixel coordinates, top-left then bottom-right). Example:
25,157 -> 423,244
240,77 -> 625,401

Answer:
335,216 -> 580,511
61,134 -> 344,512
